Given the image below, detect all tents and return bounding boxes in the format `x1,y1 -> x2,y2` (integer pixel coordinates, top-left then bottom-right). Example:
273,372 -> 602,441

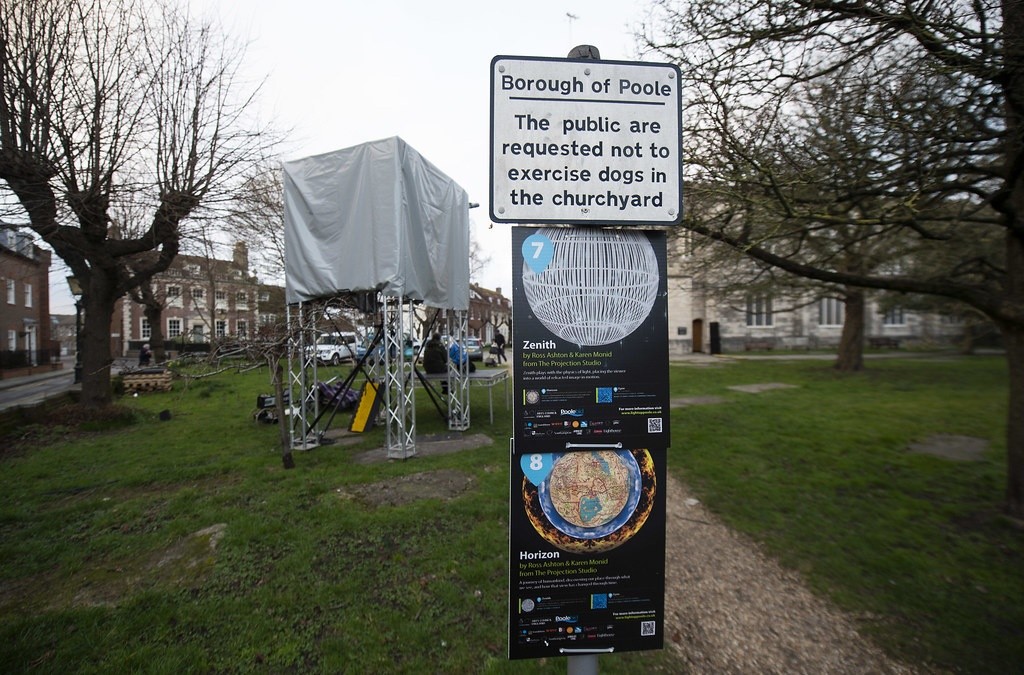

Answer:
282,136 -> 469,459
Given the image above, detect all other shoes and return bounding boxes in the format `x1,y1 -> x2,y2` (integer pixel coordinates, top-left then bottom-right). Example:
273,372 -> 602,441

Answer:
442,389 -> 454,394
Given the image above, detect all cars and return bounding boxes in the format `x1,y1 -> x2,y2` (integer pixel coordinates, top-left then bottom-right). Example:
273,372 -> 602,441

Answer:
464,338 -> 484,363
356,335 -> 395,366
399,335 -> 476,374
305,332 -> 363,366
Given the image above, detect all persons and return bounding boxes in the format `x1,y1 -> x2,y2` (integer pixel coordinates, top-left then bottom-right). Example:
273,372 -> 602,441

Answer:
448,328 -> 475,372
138,343 -> 153,366
494,329 -> 508,366
422,333 -> 455,395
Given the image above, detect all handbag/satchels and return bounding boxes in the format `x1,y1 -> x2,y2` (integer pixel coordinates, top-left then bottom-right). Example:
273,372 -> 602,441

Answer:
449,343 -> 467,364
456,361 -> 476,373
